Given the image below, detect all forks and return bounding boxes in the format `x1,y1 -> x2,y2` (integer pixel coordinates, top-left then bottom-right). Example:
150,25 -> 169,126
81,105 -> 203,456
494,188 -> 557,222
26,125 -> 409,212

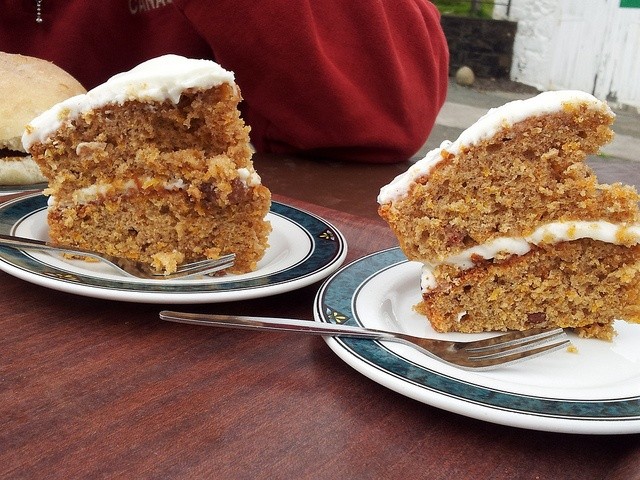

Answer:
0,234 -> 236,280
159,311 -> 571,372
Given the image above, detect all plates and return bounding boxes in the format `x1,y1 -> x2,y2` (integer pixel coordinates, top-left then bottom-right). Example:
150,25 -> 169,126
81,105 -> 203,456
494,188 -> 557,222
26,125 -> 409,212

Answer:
0,191 -> 348,305
0,182 -> 49,196
313,246 -> 640,434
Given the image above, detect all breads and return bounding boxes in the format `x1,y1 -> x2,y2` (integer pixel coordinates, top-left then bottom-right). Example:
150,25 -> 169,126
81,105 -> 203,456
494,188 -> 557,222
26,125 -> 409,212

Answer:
0,50 -> 87,187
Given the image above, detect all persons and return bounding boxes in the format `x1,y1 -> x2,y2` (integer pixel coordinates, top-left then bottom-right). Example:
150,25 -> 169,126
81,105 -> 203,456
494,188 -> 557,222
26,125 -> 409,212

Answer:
0,1 -> 450,166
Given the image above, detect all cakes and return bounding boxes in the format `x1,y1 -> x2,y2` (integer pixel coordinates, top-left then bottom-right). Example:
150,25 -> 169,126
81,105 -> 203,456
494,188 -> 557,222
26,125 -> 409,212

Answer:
21,54 -> 273,275
374,89 -> 640,341
